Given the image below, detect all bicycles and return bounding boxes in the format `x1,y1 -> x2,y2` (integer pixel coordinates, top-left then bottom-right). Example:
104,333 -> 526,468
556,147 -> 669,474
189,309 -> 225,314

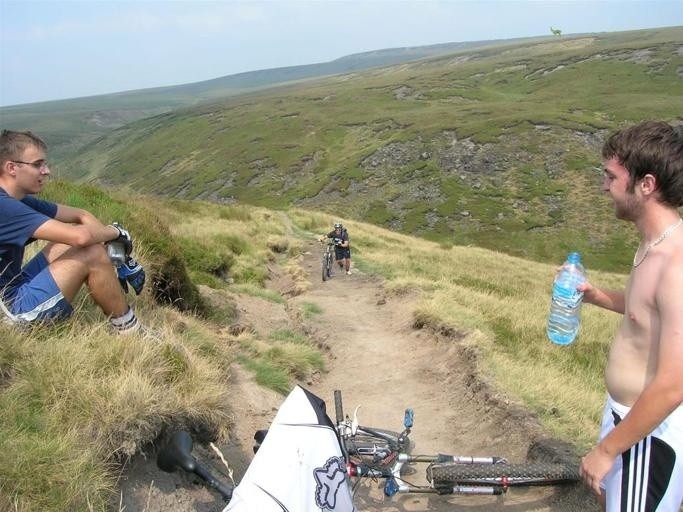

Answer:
315,236 -> 335,280
253,389 -> 587,500
148,429 -> 237,512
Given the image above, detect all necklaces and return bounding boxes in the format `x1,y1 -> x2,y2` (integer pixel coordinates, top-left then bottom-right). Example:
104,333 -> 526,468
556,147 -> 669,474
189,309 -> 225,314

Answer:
632,218 -> 682,269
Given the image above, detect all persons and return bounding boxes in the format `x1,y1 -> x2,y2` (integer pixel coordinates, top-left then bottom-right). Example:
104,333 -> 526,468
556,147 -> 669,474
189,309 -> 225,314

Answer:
319,224 -> 352,276
0,131 -> 143,335
555,121 -> 682,511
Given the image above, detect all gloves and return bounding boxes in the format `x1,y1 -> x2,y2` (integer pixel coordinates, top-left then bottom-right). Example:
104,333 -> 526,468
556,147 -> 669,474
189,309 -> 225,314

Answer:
105,222 -> 132,255
112,256 -> 146,295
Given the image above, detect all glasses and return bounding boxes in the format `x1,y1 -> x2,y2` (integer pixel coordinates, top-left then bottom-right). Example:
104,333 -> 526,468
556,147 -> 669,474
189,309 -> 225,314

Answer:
13,159 -> 44,169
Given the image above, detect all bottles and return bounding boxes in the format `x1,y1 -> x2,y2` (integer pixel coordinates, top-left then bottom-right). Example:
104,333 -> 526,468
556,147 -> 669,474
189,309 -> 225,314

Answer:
545,251 -> 586,347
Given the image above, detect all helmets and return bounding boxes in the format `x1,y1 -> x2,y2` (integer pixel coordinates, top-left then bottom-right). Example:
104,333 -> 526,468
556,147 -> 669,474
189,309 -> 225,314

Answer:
334,224 -> 342,228
